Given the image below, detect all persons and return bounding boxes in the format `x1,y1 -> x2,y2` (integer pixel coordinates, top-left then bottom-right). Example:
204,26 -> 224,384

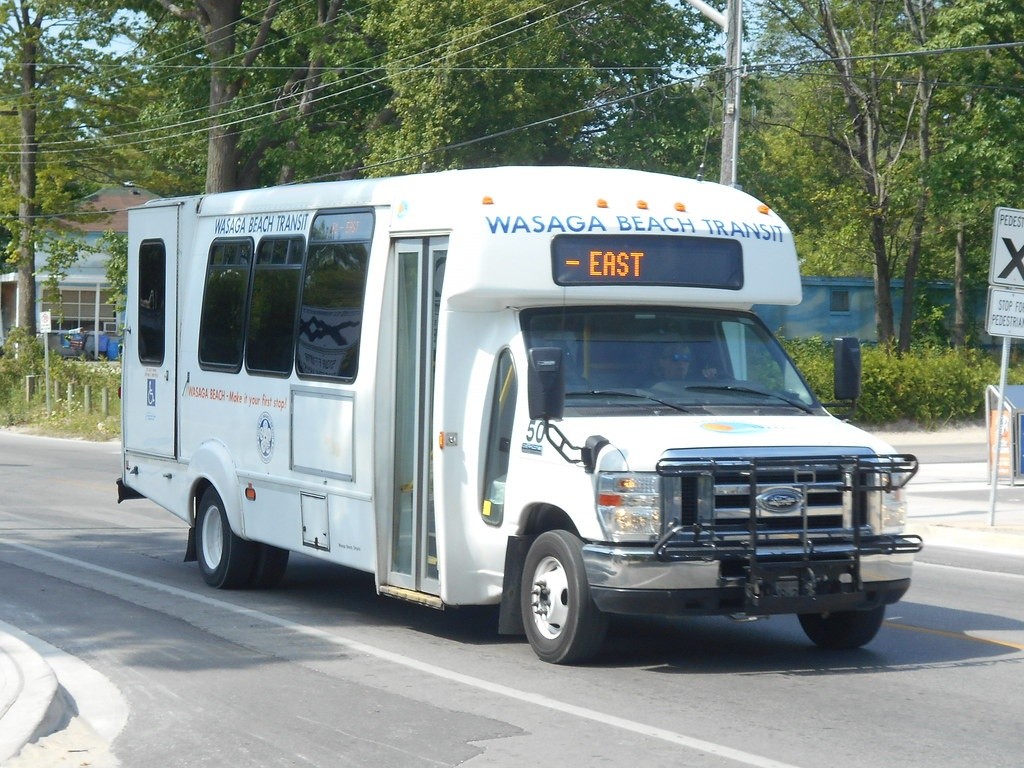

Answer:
649,343 -> 718,382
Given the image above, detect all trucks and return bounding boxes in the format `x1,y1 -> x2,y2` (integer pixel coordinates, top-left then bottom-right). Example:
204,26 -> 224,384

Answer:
115,161 -> 920,664
